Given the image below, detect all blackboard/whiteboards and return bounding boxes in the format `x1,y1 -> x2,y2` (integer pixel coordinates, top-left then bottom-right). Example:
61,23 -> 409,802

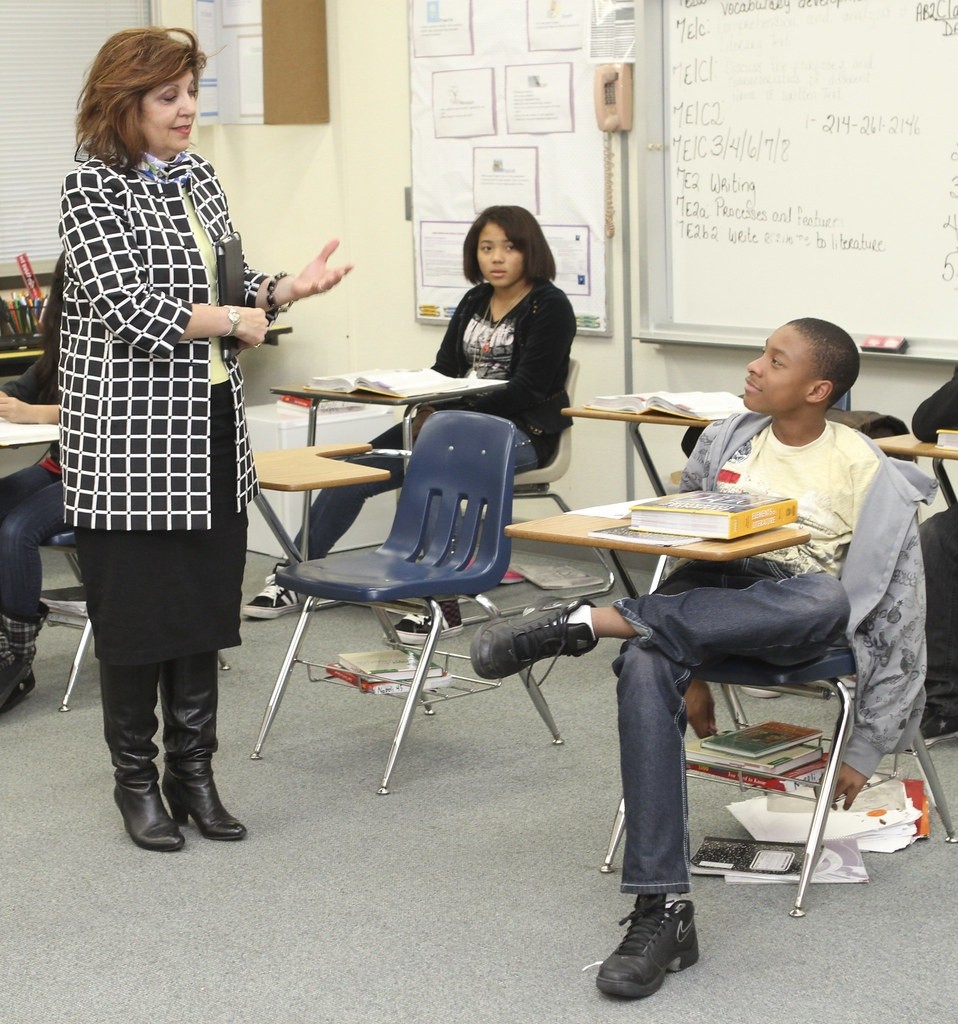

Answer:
631,0 -> 957,362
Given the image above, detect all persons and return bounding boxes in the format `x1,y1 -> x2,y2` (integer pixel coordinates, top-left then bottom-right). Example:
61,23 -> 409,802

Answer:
1,248 -> 74,712
60,25 -> 353,853
471,317 -> 940,1000
244,205 -> 577,646
897,358 -> 958,752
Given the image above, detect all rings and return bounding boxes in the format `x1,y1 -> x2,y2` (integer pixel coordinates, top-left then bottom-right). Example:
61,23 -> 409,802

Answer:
255,343 -> 261,348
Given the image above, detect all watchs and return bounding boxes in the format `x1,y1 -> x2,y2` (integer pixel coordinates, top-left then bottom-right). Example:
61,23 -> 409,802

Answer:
220,304 -> 241,337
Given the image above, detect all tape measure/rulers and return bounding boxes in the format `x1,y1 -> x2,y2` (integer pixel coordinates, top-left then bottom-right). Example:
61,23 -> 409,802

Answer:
16,253 -> 42,298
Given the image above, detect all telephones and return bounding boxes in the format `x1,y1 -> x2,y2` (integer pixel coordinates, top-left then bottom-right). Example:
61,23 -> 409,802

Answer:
593,63 -> 633,130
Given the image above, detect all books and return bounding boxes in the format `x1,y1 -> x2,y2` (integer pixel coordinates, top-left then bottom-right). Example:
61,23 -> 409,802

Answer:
936,428 -> 958,451
588,491 -> 799,547
583,390 -> 763,421
503,562 -> 606,591
275,393 -> 363,415
325,651 -> 451,696
684,721 -> 870,884
303,368 -> 471,398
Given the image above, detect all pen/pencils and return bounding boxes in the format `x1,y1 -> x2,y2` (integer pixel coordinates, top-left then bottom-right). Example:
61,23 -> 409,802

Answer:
8,292 -> 48,334
709,728 -> 718,737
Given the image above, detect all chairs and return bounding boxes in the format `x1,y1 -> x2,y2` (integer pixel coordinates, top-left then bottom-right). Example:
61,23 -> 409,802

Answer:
250,409 -> 564,796
299,354 -> 615,628
598,550 -> 956,919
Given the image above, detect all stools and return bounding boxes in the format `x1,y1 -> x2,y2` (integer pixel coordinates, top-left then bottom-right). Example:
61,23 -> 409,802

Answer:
35,529 -> 231,714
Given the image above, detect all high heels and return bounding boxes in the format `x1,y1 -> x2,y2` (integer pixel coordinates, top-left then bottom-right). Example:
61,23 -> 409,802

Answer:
162,768 -> 247,842
112,779 -> 185,851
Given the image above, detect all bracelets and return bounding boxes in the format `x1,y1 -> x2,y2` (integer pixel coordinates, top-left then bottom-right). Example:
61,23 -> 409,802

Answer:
267,272 -> 295,312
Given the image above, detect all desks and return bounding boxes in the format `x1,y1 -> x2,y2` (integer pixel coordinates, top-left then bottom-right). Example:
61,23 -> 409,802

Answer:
500,499 -> 812,564
557,405 -> 956,503
246,441 -> 388,563
270,379 -> 506,468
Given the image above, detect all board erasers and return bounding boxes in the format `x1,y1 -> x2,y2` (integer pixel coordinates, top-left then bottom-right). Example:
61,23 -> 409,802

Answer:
860,335 -> 909,354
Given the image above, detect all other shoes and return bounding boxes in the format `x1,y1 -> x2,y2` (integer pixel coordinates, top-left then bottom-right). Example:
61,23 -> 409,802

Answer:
904,715 -> 957,754
0,658 -> 36,714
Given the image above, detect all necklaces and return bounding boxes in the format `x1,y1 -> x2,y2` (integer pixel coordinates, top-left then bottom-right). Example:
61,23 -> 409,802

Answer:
470,276 -> 535,379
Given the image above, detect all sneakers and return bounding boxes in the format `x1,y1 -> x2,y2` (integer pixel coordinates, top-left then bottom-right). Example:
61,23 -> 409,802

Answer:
394,602 -> 465,645
595,895 -> 700,999
471,598 -> 601,679
243,564 -> 310,620
